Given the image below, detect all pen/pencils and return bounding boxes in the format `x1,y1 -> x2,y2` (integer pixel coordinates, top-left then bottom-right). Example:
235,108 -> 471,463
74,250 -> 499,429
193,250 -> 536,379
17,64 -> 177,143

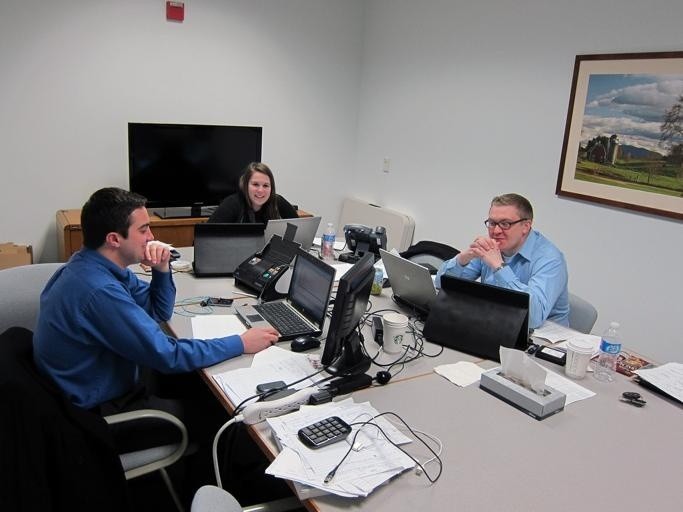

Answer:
231,290 -> 258,298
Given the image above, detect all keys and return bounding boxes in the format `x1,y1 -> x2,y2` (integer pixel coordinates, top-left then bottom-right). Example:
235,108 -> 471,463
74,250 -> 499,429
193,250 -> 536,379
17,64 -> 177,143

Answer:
619,390 -> 646,409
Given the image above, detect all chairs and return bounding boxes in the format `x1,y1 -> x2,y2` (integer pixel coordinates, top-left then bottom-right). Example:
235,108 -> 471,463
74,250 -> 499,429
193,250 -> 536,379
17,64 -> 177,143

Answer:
190,483 -> 305,512
1,263 -> 71,332
0,326 -> 189,510
405,240 -> 461,277
568,292 -> 597,334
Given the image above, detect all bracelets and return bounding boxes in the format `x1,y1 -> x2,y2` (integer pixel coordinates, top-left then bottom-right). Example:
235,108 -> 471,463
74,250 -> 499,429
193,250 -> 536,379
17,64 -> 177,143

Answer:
491,262 -> 507,272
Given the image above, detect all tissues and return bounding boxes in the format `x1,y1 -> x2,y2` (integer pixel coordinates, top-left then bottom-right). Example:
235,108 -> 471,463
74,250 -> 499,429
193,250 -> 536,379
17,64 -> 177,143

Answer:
479,344 -> 568,422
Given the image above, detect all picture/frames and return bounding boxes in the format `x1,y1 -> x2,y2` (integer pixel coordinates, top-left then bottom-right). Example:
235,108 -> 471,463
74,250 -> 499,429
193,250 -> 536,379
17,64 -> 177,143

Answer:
553,51 -> 682,224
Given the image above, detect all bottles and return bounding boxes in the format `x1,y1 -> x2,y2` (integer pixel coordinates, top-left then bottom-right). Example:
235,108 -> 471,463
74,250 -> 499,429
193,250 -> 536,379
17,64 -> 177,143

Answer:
593,322 -> 621,383
320,222 -> 334,261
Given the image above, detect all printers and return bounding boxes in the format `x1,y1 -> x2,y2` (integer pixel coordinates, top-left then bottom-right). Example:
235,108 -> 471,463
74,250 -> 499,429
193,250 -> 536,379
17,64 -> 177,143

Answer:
233,222 -> 303,301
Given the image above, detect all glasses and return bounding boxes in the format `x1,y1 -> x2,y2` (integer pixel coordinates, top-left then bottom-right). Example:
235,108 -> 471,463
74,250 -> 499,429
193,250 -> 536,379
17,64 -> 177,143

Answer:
485,218 -> 527,230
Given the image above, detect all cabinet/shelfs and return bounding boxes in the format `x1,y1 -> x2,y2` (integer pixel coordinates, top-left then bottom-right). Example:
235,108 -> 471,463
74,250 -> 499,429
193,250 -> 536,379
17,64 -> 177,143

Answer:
56,208 -> 314,261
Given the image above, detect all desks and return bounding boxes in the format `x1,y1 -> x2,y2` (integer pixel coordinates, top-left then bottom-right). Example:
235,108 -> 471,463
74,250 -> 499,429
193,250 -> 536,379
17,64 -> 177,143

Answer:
246,358 -> 683,512
123,243 -> 501,430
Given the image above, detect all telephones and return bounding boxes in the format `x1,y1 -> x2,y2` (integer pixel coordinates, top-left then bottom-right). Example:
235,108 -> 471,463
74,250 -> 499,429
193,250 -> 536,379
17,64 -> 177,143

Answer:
338,223 -> 387,266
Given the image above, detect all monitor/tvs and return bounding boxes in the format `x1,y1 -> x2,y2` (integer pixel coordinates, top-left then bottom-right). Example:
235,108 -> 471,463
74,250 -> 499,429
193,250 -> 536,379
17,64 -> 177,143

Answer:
192,222 -> 264,277
265,216 -> 323,249
321,252 -> 376,378
422,271 -> 531,363
127,121 -> 263,219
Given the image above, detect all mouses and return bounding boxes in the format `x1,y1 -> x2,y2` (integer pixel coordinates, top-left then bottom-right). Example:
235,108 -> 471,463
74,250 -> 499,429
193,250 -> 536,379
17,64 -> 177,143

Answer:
290,335 -> 321,351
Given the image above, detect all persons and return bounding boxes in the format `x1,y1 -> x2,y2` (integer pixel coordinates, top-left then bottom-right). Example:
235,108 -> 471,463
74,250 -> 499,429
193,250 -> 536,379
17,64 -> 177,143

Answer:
202,161 -> 301,225
28,187 -> 283,450
131,136 -> 214,201
428,190 -> 569,330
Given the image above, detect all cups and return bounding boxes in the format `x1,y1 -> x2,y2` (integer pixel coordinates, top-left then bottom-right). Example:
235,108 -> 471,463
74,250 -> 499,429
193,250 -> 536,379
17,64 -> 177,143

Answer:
382,313 -> 408,354
565,339 -> 593,380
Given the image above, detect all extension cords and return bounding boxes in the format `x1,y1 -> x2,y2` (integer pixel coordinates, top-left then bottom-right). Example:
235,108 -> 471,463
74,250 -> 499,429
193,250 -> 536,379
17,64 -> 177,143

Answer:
238,387 -> 318,426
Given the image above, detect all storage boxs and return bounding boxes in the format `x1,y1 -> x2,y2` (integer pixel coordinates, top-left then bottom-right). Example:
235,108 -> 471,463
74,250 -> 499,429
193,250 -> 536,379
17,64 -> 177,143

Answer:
1,243 -> 34,273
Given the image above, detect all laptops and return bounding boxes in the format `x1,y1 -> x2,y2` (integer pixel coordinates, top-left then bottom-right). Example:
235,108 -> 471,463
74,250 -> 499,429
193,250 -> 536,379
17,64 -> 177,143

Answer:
379,248 -> 439,320
233,246 -> 337,341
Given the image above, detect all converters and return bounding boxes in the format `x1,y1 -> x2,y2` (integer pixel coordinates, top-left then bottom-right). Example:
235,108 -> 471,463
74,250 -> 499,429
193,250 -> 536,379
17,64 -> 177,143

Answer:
330,373 -> 372,394
535,345 -> 568,365
310,391 -> 332,405
371,316 -> 383,343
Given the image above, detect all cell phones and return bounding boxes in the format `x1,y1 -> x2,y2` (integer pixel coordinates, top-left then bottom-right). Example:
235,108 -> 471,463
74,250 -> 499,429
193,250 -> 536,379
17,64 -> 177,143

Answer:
256,381 -> 289,395
208,296 -> 233,307
169,249 -> 180,258
